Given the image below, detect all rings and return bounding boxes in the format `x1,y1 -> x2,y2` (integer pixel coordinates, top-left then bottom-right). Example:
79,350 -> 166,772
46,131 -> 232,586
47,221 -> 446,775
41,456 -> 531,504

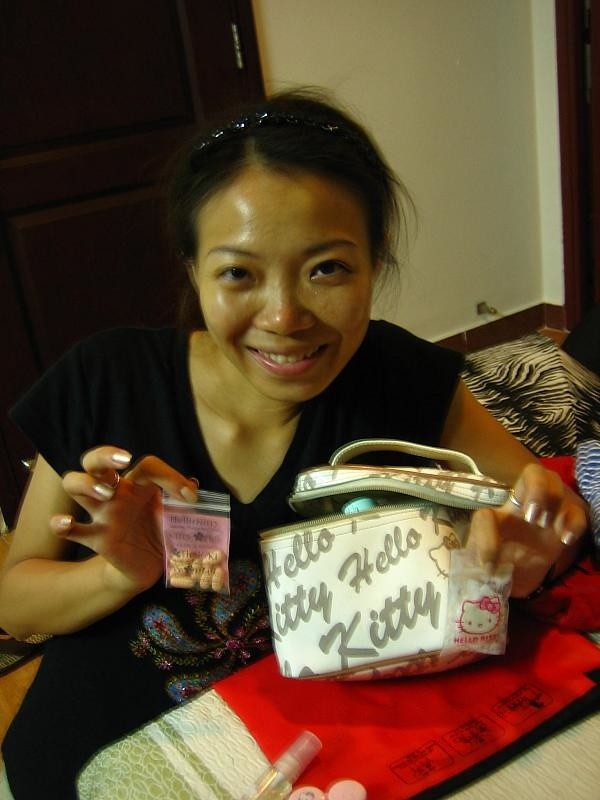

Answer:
508,487 -> 525,511
108,473 -> 119,488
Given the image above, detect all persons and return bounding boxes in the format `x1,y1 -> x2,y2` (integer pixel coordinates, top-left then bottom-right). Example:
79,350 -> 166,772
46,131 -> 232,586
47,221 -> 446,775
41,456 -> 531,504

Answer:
0,89 -> 588,800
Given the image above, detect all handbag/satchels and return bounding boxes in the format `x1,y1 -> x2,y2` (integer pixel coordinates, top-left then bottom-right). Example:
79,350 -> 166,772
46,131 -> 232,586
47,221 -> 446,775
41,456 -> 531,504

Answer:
258,440 -> 511,681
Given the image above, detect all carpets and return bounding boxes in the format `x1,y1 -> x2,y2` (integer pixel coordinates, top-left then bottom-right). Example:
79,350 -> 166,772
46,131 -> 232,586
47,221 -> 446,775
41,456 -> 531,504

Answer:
459,332 -> 600,456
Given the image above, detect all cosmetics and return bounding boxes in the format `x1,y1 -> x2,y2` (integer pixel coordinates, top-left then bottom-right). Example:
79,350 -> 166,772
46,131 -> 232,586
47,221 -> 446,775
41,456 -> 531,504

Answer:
238,730 -> 368,800
